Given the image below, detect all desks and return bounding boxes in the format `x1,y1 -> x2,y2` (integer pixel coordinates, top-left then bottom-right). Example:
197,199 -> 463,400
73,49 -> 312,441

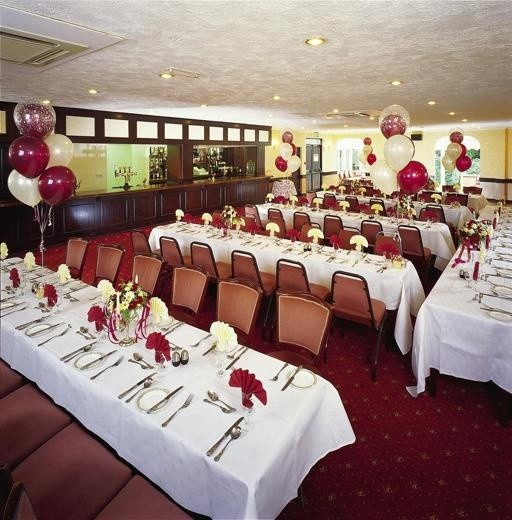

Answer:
308,188 -> 474,234
244,202 -> 456,271
149,221 -> 429,354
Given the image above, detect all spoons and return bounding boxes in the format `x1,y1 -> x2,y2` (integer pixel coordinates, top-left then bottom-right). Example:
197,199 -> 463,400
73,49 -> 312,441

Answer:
206,389 -> 236,412
213,426 -> 242,463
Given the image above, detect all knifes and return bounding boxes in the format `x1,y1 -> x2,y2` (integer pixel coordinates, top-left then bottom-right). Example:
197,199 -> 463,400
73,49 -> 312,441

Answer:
176,220 -> 388,275
206,416 -> 245,457
282,364 -> 304,392
0,260 -> 189,404
225,347 -> 249,370
147,386 -> 185,413
200,345 -> 215,358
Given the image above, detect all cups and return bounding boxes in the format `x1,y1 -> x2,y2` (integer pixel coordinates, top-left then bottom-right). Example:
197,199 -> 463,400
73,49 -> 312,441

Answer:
302,204 -> 306,211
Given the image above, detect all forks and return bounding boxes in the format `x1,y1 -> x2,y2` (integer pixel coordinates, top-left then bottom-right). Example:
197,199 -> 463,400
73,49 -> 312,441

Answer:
160,393 -> 194,428
270,362 -> 290,382
189,333 -> 212,347
225,345 -> 244,358
203,399 -> 230,414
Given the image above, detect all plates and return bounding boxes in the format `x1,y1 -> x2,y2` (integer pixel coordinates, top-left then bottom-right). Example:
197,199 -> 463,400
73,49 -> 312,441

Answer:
137,387 -> 169,412
480,206 -> 512,321
289,368 -> 317,388
263,203 -> 272,206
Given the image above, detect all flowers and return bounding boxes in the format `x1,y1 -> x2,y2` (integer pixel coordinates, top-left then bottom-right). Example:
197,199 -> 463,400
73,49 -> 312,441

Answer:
222,204 -> 237,219
102,281 -> 168,343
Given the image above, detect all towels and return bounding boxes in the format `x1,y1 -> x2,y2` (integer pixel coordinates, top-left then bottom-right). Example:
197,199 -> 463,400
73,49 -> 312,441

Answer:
43,281 -> 58,307
57,264 -> 71,285
0,242 -> 9,259
227,368 -> 268,410
9,266 -> 20,288
24,252 -> 36,271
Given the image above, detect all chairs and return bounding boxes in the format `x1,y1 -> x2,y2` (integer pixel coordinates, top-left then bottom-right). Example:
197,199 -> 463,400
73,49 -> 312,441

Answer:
374,231 -> 403,258
159,235 -> 185,278
267,208 -> 284,228
130,228 -> 168,278
323,215 -> 344,245
245,204 -> 262,227
323,270 -> 387,383
170,264 -> 210,320
241,212 -> 260,231
190,241 -> 221,279
299,222 -> 321,244
268,218 -> 286,238
275,258 -> 330,302
230,249 -> 271,328
212,209 -> 231,228
0,358 -> 192,519
361,219 -> 384,254
216,277 -> 264,348
130,254 -> 163,297
65,238 -> 90,281
334,226 -> 362,251
276,179 -> 483,229
398,224 -> 431,269
92,244 -> 125,290
293,212 -> 311,231
264,288 -> 335,379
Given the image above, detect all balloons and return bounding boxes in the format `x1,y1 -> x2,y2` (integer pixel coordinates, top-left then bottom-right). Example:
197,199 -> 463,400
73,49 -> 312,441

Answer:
274,127 -> 301,174
361,104 -> 428,197
442,127 -> 472,173
8,95 -> 77,208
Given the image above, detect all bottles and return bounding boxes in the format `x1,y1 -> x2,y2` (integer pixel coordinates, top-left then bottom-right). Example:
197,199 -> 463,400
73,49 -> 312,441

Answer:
235,166 -> 239,173
459,269 -> 464,278
472,262 -> 480,281
209,160 -> 230,174
231,166 -> 233,173
240,168 -> 242,173
194,155 -> 216,163
359,211 -> 374,220
